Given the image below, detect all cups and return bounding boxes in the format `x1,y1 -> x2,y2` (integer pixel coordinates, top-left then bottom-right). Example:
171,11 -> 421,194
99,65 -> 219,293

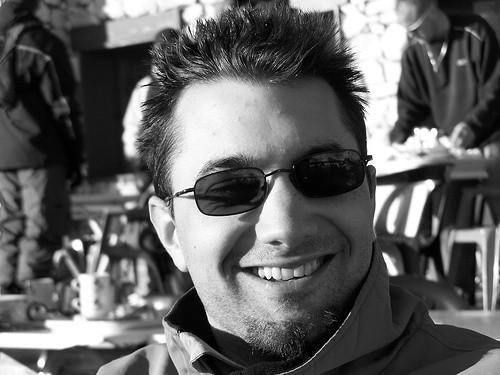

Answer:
77,272 -> 113,322
24,278 -> 58,314
1,292 -> 26,329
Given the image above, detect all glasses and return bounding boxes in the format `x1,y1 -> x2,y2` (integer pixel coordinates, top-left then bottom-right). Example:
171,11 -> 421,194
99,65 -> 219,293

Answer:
164,149 -> 374,216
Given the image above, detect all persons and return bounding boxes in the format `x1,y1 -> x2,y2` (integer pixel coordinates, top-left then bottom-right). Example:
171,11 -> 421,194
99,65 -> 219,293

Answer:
93,0 -> 500,374
389,1 -> 498,307
1,0 -> 194,300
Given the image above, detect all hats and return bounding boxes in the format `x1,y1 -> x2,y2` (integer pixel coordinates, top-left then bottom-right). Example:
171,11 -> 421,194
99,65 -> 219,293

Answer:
393,0 -> 436,32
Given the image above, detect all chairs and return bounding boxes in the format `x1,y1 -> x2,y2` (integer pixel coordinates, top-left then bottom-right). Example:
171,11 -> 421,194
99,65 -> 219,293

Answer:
440,154 -> 500,312
374,161 -> 454,282
91,208 -> 165,293
